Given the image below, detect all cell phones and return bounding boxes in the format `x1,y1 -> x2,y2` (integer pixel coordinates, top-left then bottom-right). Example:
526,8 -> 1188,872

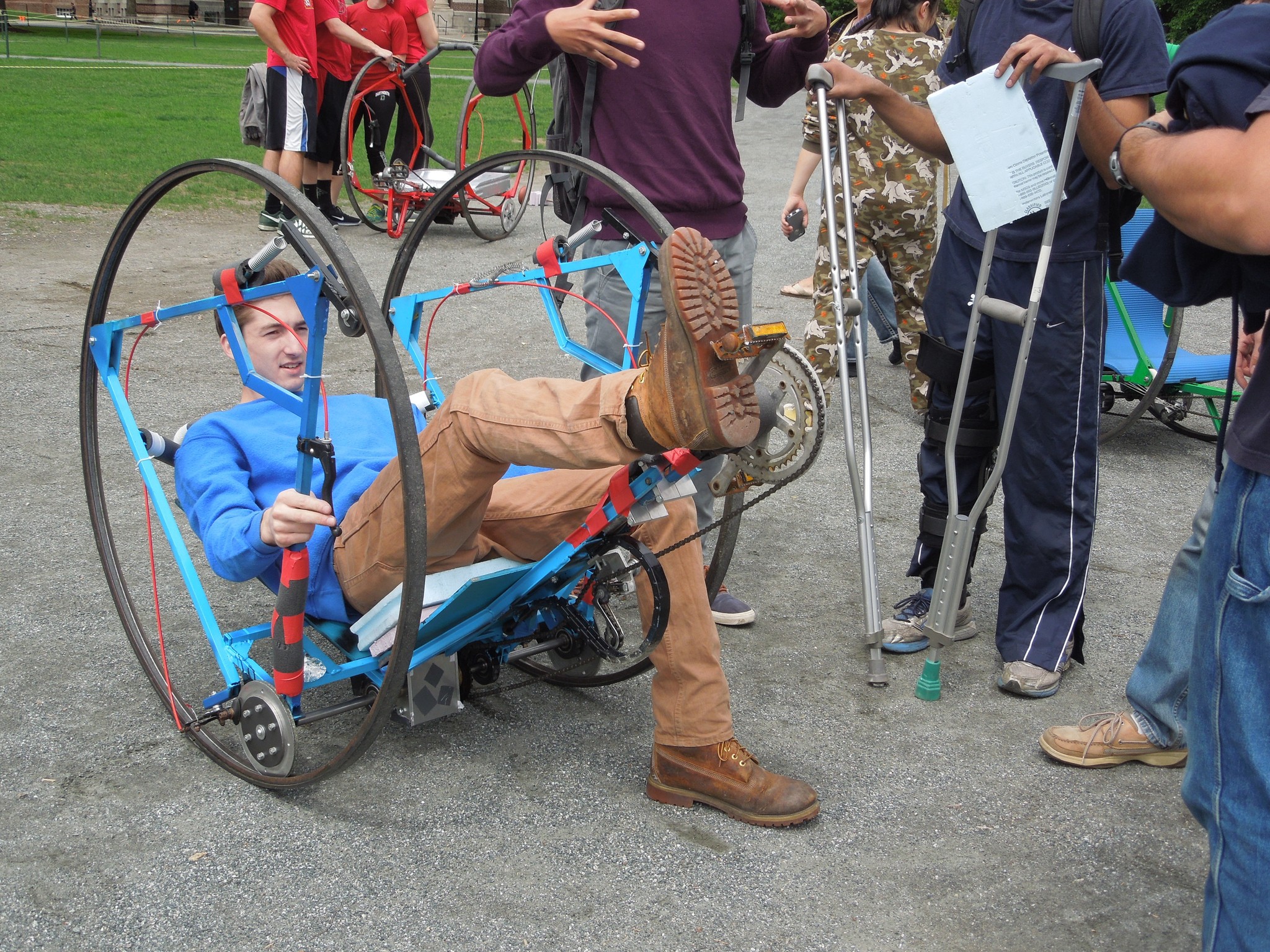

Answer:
786,207 -> 805,242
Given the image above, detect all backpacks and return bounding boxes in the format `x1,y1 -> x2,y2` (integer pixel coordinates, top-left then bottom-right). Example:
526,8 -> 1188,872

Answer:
544,0 -> 757,225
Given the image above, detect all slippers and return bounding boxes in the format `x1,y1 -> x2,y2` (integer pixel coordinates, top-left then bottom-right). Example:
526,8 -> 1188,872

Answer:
779,281 -> 813,299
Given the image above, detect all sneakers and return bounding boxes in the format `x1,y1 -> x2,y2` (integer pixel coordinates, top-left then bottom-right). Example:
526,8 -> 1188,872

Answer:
313,207 -> 339,230
627,228 -> 759,452
996,630 -> 1074,697
365,203 -> 386,223
1037,708 -> 1187,769
881,588 -> 976,653
277,213 -> 316,239
645,737 -> 820,826
258,203 -> 284,231
835,361 -> 857,378
889,340 -> 903,365
321,203 -> 361,226
703,565 -> 756,625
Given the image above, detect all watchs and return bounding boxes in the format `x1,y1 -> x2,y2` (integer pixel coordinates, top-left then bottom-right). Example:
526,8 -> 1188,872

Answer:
1108,119 -> 1169,192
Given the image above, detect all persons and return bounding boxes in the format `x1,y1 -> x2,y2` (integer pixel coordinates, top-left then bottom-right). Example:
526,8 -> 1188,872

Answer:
1037,310 -> 1269,772
777,0 -> 964,429
828,0 -> 904,381
247,0 -> 321,238
386,0 -> 439,222
301,0 -> 396,230
796,0 -> 1176,702
69,3 -> 79,21
331,0 -> 409,226
188,0 -> 200,24
782,275 -> 814,300
1103,55 -> 1270,952
171,226 -> 827,830
469,0 -> 837,628
